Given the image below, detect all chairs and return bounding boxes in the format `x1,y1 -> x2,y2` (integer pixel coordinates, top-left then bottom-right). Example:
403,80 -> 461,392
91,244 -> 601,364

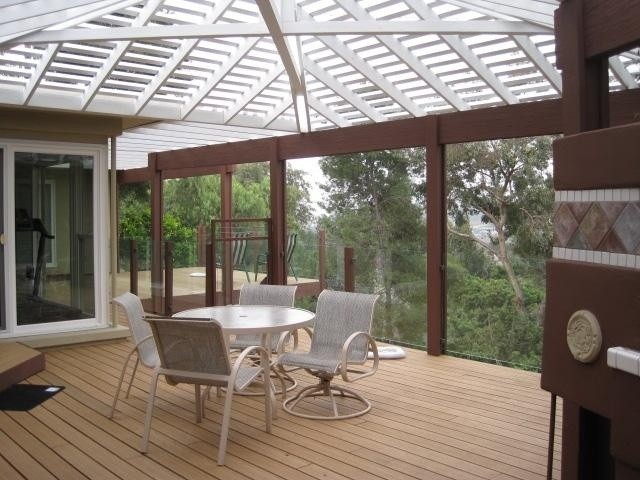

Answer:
275,289 -> 382,422
217,231 -> 299,279
221,283 -> 316,374
107,292 -> 211,421
138,314 -> 274,467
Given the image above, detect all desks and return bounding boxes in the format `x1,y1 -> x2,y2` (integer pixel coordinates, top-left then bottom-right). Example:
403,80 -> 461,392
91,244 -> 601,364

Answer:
170,304 -> 316,396
